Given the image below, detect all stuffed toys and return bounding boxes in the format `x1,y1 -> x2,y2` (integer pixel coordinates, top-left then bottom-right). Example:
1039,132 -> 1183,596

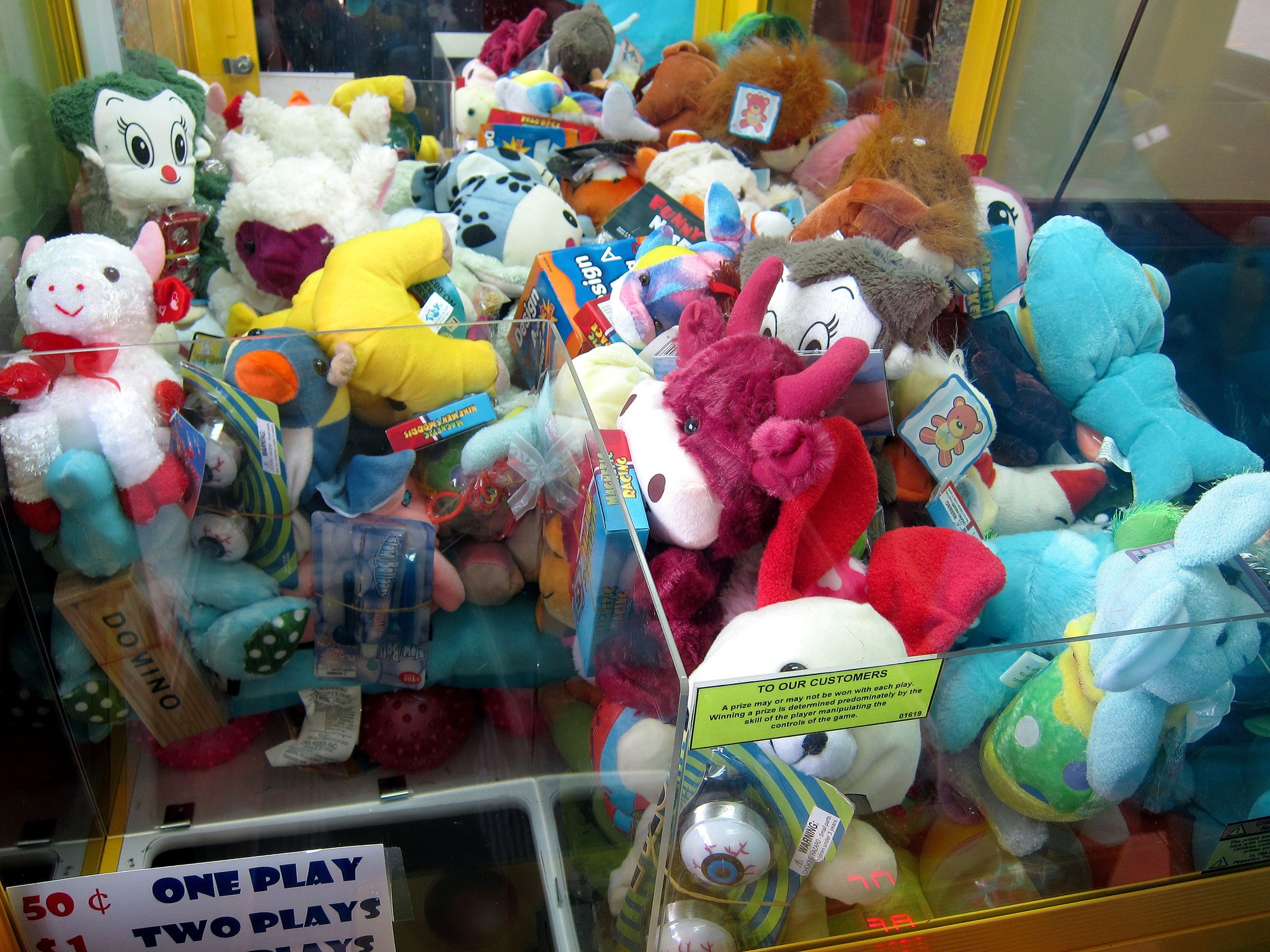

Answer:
0,1 -> 1270,952
